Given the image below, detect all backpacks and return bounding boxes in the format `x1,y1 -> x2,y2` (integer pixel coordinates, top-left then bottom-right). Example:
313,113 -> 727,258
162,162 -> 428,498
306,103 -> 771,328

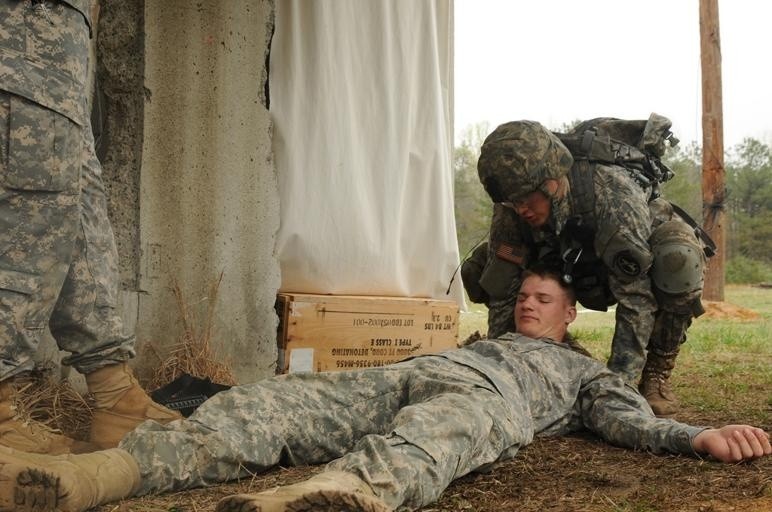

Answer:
552,112 -> 680,288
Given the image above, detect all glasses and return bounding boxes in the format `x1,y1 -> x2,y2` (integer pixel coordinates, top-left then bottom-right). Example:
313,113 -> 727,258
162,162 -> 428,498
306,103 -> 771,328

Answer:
500,194 -> 538,207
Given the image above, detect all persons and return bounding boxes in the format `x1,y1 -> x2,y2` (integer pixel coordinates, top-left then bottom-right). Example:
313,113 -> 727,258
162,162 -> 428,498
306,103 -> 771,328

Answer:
0,2 -> 191,459
457,111 -> 710,420
1,258 -> 772,507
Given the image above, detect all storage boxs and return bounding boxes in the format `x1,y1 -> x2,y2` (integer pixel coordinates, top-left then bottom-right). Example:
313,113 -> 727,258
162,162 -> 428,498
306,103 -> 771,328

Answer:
277,291 -> 460,373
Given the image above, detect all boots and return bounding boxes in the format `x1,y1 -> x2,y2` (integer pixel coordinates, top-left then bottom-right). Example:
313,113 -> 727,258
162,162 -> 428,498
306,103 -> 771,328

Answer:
562,330 -> 592,358
638,351 -> 679,420
89,361 -> 187,451
216,472 -> 388,511
0,384 -> 141,512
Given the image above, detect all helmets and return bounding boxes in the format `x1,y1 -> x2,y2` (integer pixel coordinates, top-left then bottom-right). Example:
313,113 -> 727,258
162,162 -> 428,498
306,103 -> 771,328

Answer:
477,120 -> 574,203
